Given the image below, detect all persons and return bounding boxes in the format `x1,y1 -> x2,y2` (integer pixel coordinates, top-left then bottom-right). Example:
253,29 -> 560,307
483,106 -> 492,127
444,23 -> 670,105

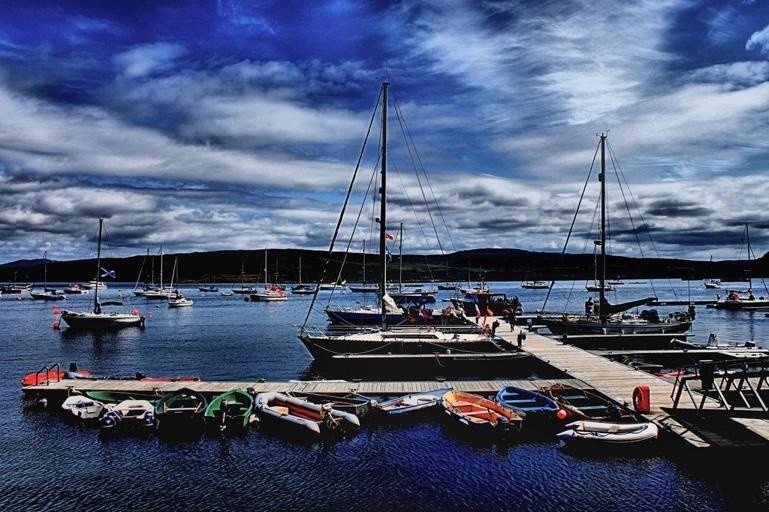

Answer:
440,301 -> 467,326
583,296 -> 600,324
518,330 -> 523,350
502,306 -> 514,324
509,314 -> 515,332
481,318 -> 501,340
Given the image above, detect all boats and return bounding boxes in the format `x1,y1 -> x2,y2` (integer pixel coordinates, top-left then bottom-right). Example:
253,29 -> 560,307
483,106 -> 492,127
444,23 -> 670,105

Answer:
61,386 -> 165,430
494,386 -> 560,426
203,388 -> 255,433
520,280 -> 551,289
374,387 -> 453,422
253,390 -> 361,441
554,420 -> 658,450
63,368 -> 201,382
547,382 -> 637,422
284,389 -> 369,421
153,388 -> 207,427
64,278 -> 108,294
668,332 -> 759,350
440,390 -> 523,436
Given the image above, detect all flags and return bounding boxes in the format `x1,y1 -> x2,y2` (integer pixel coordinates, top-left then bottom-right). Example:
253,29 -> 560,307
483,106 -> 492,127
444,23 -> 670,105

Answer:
386,232 -> 394,240
100,267 -> 117,280
386,247 -> 392,262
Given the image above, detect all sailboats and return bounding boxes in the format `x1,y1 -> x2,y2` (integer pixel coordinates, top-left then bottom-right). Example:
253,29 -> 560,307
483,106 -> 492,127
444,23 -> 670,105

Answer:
29,251 -> 66,300
59,217 -> 145,330
585,244 -> 625,292
320,221 -> 523,329
295,74 -> 507,361
535,128 -> 697,335
716,223 -> 767,309
133,244 -> 194,307
703,254 -> 722,289
198,249 -> 318,301
